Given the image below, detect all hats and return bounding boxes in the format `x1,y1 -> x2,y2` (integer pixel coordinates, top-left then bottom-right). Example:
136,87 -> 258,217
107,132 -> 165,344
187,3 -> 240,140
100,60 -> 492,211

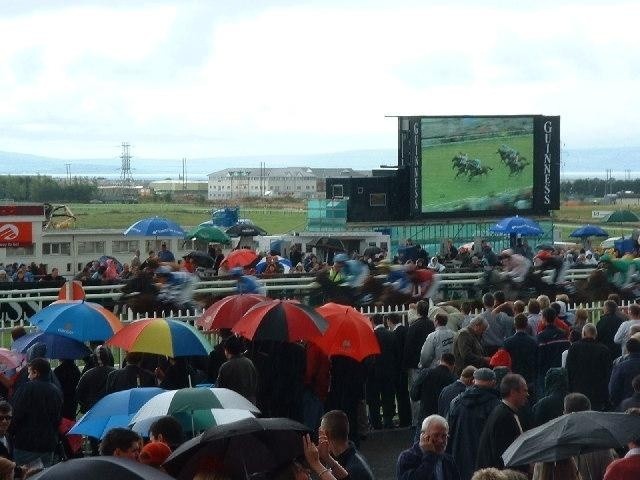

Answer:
473,368 -> 496,379
140,442 -> 172,464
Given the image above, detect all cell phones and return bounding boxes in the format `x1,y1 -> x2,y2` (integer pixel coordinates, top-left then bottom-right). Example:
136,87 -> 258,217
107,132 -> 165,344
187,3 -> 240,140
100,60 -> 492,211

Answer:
421,431 -> 430,441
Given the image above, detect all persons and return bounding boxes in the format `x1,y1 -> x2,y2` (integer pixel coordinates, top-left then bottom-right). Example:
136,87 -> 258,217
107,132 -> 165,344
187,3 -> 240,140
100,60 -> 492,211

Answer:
457,144 -> 522,173
1,229 -> 640,479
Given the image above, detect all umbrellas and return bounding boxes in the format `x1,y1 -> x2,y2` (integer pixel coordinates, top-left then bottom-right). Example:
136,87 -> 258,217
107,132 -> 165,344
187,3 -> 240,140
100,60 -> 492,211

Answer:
225,224 -> 267,249
121,215 -> 186,256
488,215 -> 544,246
600,208 -> 640,240
568,224 -> 609,250
182,224 -> 231,255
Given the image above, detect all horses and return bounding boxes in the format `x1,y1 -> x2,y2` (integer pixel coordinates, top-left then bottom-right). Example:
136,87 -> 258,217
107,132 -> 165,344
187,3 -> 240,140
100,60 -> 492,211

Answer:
451,151 -> 494,182
494,144 -> 530,178
